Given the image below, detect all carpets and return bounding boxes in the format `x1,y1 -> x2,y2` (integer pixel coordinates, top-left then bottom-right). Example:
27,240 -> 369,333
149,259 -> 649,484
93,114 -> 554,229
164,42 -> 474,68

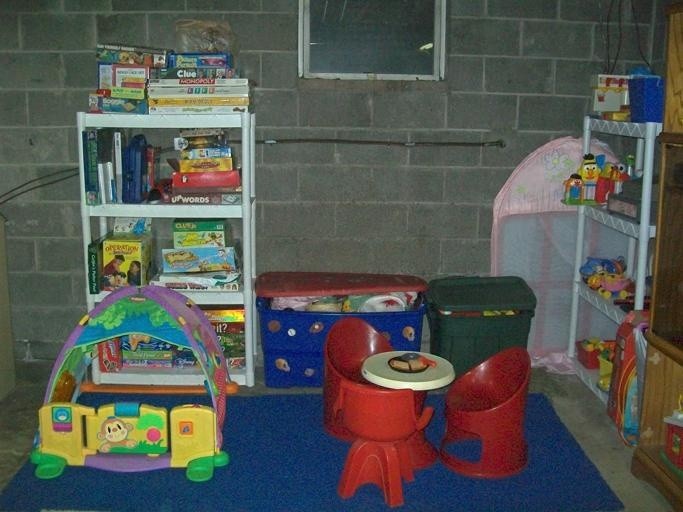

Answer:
2,392 -> 625,512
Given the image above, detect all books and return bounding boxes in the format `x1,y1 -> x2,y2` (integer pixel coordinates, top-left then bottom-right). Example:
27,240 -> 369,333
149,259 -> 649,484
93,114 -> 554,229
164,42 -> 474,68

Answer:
83,129 -> 160,208
147,78 -> 254,112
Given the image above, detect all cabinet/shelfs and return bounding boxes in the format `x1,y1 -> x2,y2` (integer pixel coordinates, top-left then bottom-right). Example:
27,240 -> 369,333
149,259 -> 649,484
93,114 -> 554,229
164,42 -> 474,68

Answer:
565,1 -> 683,511
76,105 -> 258,388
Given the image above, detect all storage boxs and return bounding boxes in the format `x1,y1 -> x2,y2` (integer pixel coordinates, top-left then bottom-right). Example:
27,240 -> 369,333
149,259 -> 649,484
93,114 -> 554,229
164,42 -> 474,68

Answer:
256,267 -> 537,389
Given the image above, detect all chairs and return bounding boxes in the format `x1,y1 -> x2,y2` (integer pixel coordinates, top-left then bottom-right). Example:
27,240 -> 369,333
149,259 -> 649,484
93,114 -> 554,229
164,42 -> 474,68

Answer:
320,318 -> 533,508
